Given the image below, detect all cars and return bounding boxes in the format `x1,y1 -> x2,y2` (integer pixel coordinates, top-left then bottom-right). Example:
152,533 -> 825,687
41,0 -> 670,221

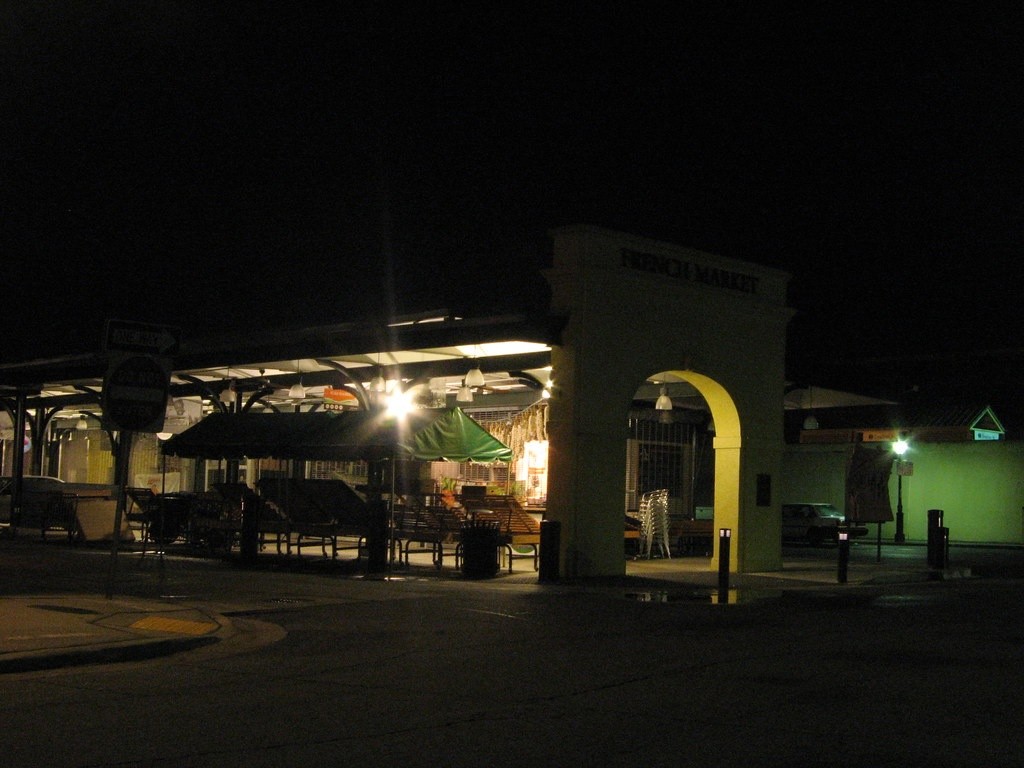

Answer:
0,476 -> 67,527
780,503 -> 870,545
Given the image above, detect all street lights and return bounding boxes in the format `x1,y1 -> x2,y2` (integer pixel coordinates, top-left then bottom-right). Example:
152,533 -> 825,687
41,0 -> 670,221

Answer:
76,410 -> 120,600
893,440 -> 906,545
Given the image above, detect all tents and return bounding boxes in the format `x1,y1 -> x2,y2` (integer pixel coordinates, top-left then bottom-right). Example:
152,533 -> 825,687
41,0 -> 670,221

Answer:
160,402 -> 510,573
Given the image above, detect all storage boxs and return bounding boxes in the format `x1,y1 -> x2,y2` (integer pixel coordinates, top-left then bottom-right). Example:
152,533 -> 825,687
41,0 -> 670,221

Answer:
695,507 -> 714,520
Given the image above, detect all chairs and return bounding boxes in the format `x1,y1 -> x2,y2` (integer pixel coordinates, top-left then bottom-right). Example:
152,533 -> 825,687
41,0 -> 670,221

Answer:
638,488 -> 672,561
35,488 -> 539,575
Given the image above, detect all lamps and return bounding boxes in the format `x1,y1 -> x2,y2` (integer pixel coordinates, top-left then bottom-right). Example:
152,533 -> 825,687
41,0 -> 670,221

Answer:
803,387 -> 820,430
456,344 -> 488,402
76,404 -> 88,430
654,373 -> 675,425
219,353 -> 386,403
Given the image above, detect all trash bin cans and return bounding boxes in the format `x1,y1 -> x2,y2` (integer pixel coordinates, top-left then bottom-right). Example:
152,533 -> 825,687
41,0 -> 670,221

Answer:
456,518 -> 502,580
